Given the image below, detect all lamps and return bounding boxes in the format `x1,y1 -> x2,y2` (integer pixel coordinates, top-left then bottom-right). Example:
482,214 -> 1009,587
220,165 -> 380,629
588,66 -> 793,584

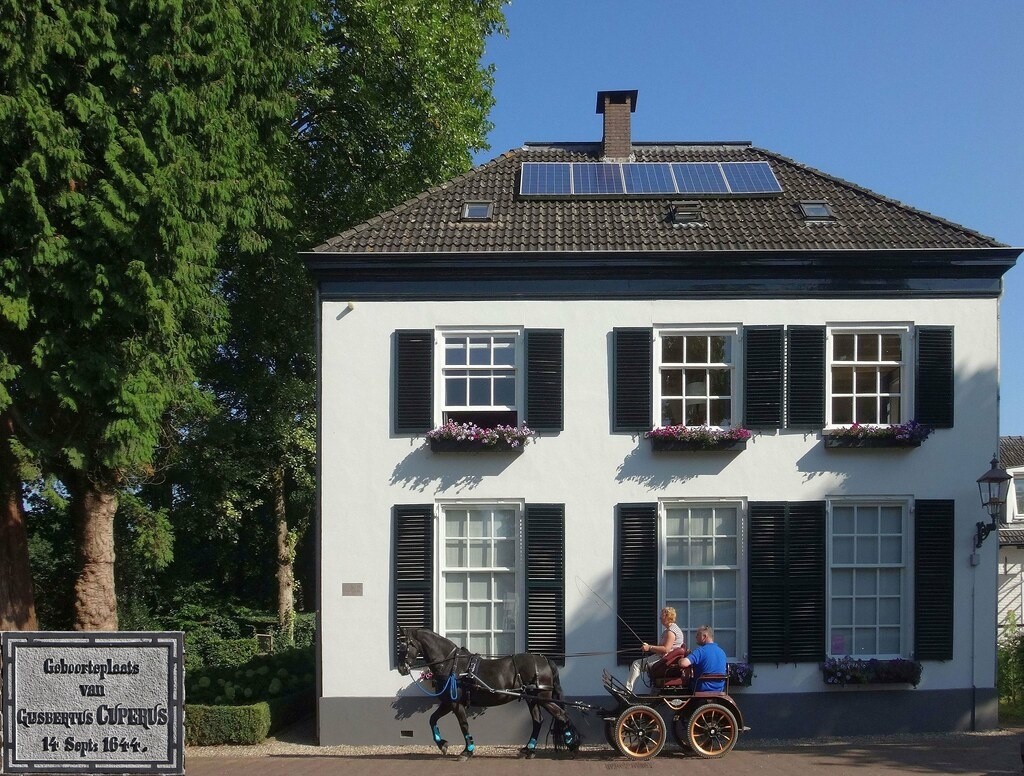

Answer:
975,453 -> 1013,549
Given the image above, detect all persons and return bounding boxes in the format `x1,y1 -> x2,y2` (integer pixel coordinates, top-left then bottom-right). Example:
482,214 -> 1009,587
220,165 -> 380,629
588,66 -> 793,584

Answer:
678,625 -> 727,691
625,607 -> 684,694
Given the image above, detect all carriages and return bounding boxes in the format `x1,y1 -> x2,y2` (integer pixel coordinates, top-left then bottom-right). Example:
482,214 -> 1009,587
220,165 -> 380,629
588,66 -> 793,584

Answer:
393,623 -> 750,763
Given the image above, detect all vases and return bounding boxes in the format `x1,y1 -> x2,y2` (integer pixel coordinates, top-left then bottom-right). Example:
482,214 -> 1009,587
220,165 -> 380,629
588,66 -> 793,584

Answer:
650,440 -> 746,451
729,676 -> 751,687
429,436 -> 526,452
823,673 -> 914,684
823,437 -> 921,449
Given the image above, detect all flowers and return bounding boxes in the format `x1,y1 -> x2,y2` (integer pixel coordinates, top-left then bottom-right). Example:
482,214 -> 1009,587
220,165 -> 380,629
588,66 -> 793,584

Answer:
727,662 -> 758,688
643,424 -> 752,448
822,654 -> 924,685
423,418 -> 535,453
421,669 -> 436,681
828,418 -> 932,447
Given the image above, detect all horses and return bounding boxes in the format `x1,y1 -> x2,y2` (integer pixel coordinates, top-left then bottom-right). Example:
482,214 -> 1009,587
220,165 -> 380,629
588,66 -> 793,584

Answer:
394,624 -> 587,763
